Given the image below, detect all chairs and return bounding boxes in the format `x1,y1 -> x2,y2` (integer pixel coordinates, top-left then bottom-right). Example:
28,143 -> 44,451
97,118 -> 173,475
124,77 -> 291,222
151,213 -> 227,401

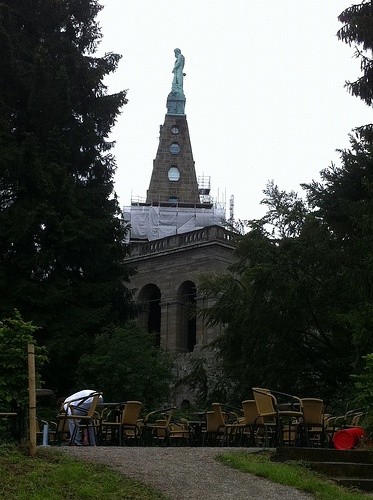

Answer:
34,385 -> 368,446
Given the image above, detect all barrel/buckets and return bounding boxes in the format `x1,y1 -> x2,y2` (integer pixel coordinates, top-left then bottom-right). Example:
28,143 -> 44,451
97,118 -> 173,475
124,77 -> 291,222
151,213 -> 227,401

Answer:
334,428 -> 364,450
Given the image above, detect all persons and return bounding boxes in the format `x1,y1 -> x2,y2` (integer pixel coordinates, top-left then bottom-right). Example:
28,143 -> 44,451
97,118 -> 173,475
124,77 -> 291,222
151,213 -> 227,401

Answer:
57,389 -> 104,446
171,48 -> 184,90
